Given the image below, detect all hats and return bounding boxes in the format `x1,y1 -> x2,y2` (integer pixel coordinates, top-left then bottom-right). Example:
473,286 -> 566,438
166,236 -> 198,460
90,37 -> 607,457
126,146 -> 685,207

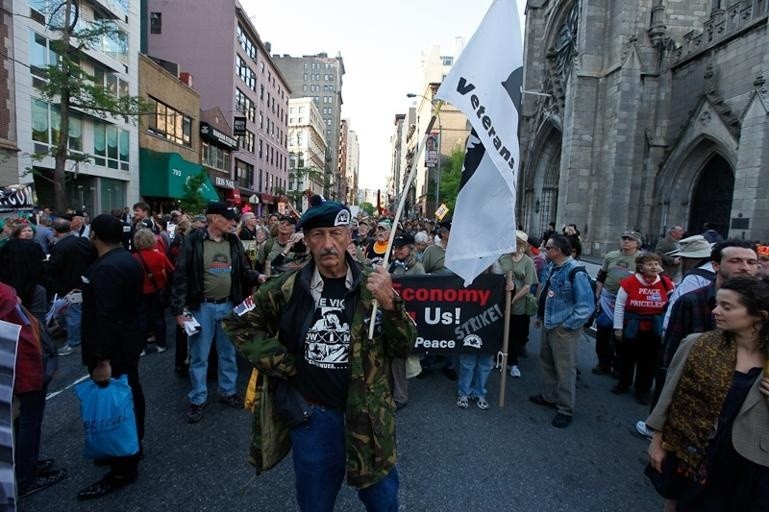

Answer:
392,232 -> 415,246
206,201 -> 239,217
621,230 -> 642,248
295,200 -> 352,229
663,234 -> 717,258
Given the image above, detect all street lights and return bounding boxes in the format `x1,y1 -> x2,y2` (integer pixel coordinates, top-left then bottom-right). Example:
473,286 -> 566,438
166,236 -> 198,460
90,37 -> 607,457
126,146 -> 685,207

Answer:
408,93 -> 441,213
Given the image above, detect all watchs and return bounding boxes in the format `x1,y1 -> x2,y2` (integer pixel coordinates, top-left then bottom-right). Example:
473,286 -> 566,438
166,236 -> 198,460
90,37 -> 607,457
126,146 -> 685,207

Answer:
75,214 -> 146,501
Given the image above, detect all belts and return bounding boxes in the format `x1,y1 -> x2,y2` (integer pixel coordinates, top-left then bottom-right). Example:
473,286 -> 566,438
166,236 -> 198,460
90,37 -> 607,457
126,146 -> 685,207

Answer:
201,295 -> 231,304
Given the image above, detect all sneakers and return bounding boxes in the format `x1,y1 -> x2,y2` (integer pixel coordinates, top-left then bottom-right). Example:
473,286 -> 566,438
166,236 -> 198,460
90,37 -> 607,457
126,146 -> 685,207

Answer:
471,396 -> 490,410
57,342 -> 74,356
519,347 -> 527,357
633,419 -> 656,442
213,393 -> 244,409
529,395 -> 559,410
17,459 -> 66,498
551,413 -> 572,428
507,365 -> 521,377
455,396 -> 470,408
592,363 -> 649,406
441,365 -> 458,381
187,402 -> 207,424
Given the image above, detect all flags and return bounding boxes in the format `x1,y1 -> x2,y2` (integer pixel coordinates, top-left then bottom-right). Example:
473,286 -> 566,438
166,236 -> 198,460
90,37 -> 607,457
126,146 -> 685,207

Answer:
433,0 -> 524,291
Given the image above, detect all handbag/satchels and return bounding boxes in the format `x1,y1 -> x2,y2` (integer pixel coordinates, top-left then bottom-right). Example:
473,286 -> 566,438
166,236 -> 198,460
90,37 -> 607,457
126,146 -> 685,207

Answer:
643,462 -> 692,499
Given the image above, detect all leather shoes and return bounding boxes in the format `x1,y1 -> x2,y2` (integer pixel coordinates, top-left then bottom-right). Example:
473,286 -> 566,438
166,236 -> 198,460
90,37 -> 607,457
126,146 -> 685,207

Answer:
77,476 -> 127,501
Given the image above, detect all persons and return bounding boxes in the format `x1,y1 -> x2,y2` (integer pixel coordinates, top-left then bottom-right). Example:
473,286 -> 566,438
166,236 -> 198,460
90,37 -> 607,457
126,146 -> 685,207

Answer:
0,239 -> 66,496
644,277 -> 769,511
347,212 -> 596,429
2,204 -> 309,423
591,225 -> 768,436
222,201 -> 418,511
